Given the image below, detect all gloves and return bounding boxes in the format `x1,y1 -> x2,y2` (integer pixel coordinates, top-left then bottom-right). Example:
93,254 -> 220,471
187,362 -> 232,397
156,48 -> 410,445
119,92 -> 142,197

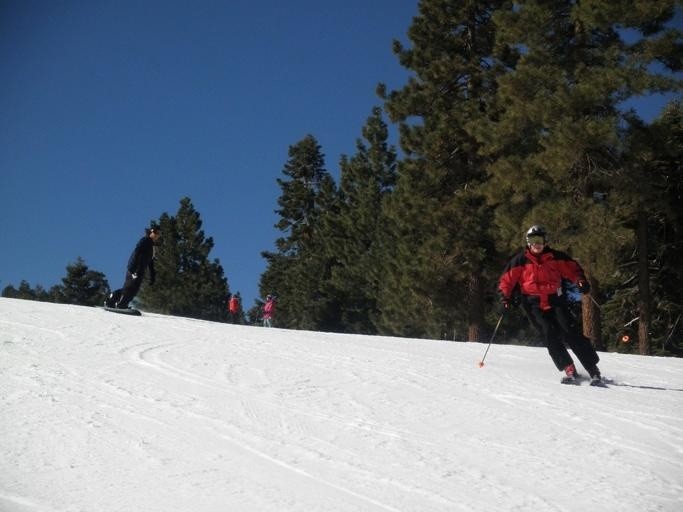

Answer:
579,281 -> 590,295
498,299 -> 509,315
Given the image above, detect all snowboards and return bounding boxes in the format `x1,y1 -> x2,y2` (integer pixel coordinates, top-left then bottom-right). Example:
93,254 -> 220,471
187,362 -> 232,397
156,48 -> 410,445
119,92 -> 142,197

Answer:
104,302 -> 141,316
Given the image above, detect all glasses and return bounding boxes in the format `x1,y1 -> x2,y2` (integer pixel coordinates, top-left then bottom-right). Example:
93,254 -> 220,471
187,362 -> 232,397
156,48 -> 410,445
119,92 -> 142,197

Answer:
526,235 -> 544,245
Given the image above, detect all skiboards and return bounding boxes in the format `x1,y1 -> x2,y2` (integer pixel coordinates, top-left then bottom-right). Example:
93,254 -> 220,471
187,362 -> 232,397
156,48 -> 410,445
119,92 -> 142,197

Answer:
559,373 -> 604,388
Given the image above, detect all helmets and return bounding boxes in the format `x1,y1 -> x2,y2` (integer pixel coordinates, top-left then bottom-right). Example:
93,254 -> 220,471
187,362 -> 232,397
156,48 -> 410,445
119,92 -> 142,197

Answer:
525,225 -> 547,249
266,294 -> 272,300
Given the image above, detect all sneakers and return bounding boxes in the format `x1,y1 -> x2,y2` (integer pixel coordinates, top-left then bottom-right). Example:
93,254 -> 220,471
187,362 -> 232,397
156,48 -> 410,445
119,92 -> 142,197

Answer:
589,365 -> 601,381
104,298 -> 130,308
564,363 -> 578,380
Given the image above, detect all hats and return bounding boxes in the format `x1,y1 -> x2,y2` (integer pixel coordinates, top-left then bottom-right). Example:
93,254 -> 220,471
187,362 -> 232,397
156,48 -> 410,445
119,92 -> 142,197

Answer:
150,221 -> 161,233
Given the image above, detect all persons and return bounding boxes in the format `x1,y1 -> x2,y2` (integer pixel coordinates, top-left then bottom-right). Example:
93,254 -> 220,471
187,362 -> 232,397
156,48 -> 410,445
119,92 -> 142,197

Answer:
104,224 -> 164,311
496,225 -> 604,386
260,293 -> 274,328
229,295 -> 241,324
